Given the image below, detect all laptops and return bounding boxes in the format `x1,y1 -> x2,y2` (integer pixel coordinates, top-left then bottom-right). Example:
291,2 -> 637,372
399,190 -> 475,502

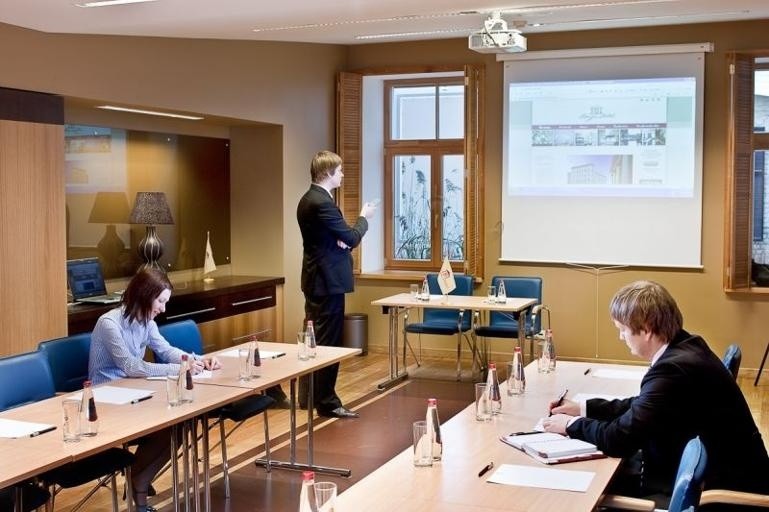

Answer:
66,256 -> 125,306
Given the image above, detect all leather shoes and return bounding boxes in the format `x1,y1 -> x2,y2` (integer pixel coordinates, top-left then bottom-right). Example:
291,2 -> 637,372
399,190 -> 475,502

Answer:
300,403 -> 359,417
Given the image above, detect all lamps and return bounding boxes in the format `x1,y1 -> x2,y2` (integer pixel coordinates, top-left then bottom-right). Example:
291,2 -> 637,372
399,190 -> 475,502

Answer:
87,191 -> 130,278
130,191 -> 175,274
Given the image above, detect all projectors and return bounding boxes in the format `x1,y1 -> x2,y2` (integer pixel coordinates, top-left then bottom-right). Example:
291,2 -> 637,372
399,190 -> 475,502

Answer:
468,32 -> 526,55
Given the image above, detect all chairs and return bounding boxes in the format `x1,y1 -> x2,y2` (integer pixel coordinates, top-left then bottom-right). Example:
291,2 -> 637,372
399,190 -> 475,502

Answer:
0,351 -> 135,512
152,320 -> 271,497
596,435 -> 708,512
723,344 -> 741,380
38,333 -> 141,500
471,276 -> 551,382
0,483 -> 51,512
403,272 -> 483,382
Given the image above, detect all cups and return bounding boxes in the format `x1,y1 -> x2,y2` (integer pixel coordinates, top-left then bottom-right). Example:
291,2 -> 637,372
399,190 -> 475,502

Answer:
538,340 -> 550,374
314,481 -> 337,512
413,420 -> 434,467
239,348 -> 253,379
410,284 -> 418,301
297,332 -> 309,361
507,361 -> 522,397
487,285 -> 496,305
62,398 -> 82,443
475,382 -> 493,420
166,371 -> 182,406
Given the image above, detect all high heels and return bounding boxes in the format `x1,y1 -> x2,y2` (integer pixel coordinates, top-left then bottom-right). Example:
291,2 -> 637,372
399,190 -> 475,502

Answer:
122,476 -> 158,512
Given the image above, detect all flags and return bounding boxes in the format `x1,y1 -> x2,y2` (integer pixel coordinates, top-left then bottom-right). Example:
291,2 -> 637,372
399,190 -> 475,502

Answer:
203,238 -> 217,274
437,256 -> 457,295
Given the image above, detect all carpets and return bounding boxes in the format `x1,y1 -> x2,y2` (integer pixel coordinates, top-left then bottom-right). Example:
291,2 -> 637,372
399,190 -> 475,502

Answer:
121,376 -> 506,511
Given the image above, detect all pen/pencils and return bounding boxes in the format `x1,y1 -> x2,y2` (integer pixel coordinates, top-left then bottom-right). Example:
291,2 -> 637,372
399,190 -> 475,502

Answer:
272,353 -> 286,359
131,396 -> 153,404
584,367 -> 591,375
509,431 -> 544,436
192,351 -> 203,372
479,462 -> 493,477
548,389 -> 569,417
30,426 -> 57,437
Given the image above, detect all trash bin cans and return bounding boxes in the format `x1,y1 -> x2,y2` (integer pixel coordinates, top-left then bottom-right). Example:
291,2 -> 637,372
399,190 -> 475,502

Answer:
344,312 -> 368,356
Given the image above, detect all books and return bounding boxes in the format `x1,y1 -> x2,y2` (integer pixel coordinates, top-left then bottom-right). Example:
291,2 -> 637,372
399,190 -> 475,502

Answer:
147,369 -> 212,381
499,430 -> 607,465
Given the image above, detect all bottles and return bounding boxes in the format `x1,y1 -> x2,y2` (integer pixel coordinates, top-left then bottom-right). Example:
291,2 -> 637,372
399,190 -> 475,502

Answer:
512,345 -> 526,392
305,320 -> 317,358
299,471 -> 319,512
498,279 -> 506,303
486,361 -> 501,415
425,398 -> 443,463
180,354 -> 195,404
81,381 -> 99,436
249,335 -> 262,379
545,329 -> 557,371
421,275 -> 430,302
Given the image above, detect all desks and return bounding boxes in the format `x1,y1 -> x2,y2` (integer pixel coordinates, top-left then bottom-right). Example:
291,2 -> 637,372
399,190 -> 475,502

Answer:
154,339 -> 363,511
0,378 -> 255,512
317,358 -> 650,512
371,293 -> 539,393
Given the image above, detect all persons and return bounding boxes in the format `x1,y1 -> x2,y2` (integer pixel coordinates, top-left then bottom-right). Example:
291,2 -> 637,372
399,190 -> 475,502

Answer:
296,149 -> 378,418
543,280 -> 769,512
88,268 -> 222,512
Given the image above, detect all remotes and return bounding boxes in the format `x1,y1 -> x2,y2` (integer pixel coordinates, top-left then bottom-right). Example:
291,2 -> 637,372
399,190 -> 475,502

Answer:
369,198 -> 383,207
